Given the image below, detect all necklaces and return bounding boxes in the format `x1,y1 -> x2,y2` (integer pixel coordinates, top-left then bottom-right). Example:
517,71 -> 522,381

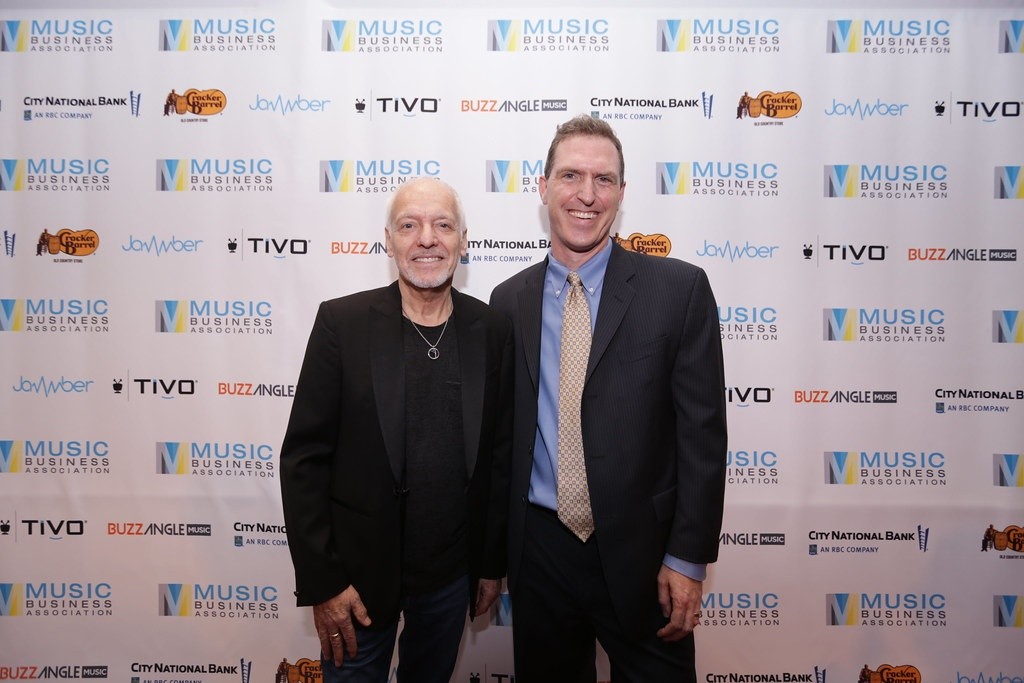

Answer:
399,296 -> 452,360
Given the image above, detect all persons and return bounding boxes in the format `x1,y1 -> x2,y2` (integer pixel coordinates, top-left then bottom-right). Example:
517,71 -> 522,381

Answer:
486,113 -> 728,682
280,175 -> 501,683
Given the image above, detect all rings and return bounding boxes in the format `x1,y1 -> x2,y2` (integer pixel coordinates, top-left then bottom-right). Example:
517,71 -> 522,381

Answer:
693,611 -> 703,618
328,632 -> 341,638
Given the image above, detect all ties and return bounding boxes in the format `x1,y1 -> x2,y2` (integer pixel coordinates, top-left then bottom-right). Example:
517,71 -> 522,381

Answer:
557,273 -> 597,542
